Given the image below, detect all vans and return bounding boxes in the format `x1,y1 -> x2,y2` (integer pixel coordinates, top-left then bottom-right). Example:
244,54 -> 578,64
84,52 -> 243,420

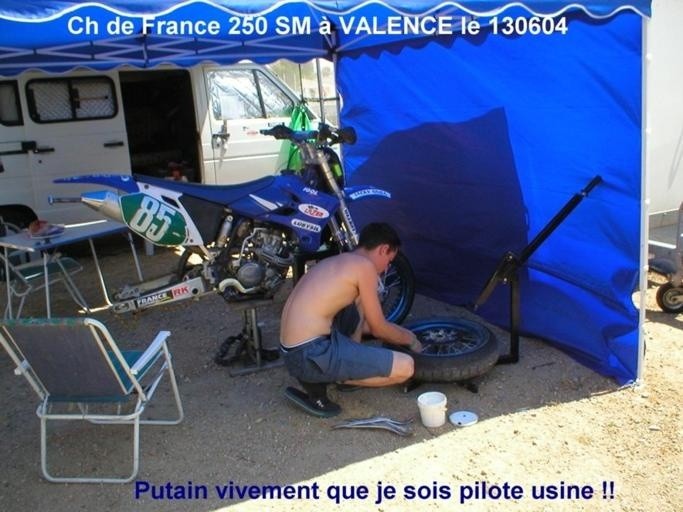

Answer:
0,55 -> 348,282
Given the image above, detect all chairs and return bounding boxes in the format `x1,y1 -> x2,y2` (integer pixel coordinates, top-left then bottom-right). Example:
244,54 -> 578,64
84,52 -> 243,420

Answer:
0,216 -> 87,322
0,319 -> 184,484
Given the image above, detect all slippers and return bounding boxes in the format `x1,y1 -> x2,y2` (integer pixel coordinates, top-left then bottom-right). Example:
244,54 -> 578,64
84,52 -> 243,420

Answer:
285,386 -> 341,418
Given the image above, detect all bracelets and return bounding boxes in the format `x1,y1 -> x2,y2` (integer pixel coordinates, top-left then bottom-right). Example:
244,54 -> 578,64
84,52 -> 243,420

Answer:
408,334 -> 418,349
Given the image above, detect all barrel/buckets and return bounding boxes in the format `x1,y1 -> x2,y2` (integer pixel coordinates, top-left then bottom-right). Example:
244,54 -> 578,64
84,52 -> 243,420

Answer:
418,392 -> 448,427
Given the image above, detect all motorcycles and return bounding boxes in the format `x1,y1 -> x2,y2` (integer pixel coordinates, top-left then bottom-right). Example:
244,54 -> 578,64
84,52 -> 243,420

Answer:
43,114 -> 419,341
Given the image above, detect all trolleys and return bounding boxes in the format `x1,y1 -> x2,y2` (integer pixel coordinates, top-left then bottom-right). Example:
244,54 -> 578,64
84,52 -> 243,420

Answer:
647,201 -> 683,313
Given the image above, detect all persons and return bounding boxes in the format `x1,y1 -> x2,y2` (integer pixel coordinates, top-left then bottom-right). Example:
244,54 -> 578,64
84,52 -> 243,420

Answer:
280,222 -> 423,418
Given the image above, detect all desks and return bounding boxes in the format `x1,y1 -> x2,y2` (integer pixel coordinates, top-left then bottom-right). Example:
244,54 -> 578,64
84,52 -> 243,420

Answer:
0,216 -> 144,318
